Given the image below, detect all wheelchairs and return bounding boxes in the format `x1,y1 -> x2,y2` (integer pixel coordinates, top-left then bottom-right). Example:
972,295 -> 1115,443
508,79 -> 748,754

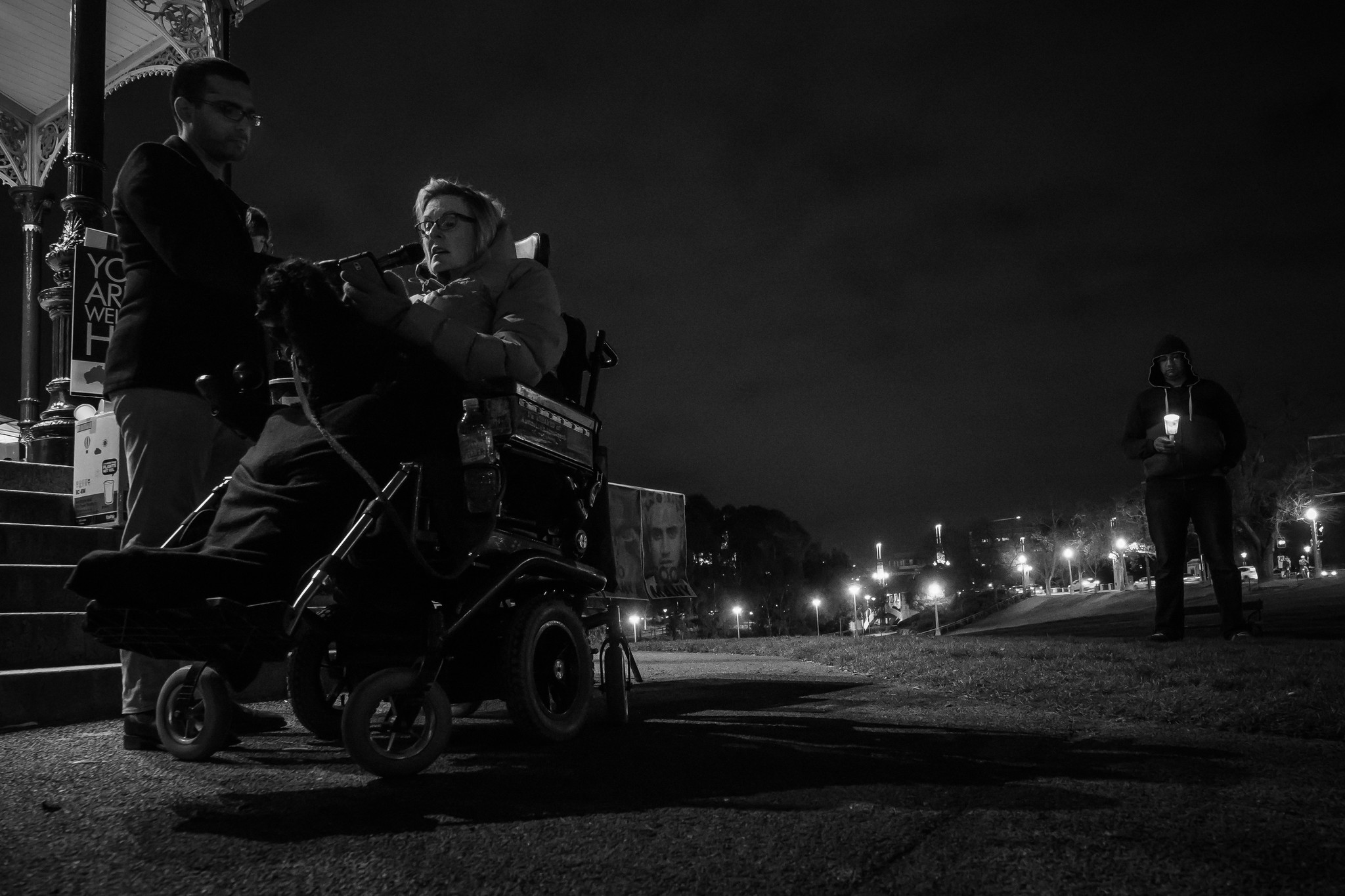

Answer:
59,226 -> 644,785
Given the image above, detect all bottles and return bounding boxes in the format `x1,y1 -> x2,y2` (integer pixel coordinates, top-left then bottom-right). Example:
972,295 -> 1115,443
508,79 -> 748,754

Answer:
457,398 -> 504,514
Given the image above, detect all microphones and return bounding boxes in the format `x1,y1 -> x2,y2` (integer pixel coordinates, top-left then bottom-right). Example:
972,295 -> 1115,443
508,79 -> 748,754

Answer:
378,242 -> 426,269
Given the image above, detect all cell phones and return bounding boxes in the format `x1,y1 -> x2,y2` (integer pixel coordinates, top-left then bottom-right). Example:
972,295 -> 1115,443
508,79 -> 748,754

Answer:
337,251 -> 389,294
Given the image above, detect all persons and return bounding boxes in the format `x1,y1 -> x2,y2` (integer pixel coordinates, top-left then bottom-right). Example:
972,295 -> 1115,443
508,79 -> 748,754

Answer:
103,56 -> 288,752
1282,556 -> 1291,579
202,174 -> 568,608
1126,335 -> 1258,641
1298,555 -> 1311,578
641,490 -> 693,598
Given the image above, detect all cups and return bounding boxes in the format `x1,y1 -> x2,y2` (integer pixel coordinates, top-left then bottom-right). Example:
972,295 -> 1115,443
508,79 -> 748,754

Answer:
1163,415 -> 1180,435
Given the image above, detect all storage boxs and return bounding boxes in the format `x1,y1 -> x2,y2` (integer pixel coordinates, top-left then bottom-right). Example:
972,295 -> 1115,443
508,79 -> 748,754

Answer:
72,411 -> 129,528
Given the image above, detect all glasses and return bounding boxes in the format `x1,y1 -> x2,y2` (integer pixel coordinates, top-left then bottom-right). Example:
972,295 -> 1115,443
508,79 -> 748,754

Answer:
188,96 -> 263,126
415,211 -> 479,238
1157,354 -> 1181,363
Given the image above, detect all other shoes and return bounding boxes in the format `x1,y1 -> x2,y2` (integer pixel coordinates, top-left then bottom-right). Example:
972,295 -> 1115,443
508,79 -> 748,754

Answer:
122,711 -> 241,751
1150,623 -> 1178,641
192,702 -> 289,730
1228,631 -> 1251,643
